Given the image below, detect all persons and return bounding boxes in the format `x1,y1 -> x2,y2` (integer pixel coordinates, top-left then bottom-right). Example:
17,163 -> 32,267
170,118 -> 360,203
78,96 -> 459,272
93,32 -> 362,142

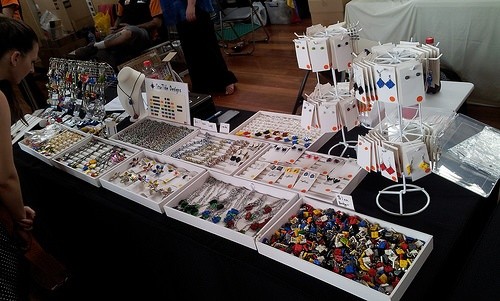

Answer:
0,0 -> 20,19
76,0 -> 162,64
116,66 -> 145,121
165,0 -> 240,96
0,17 -> 39,301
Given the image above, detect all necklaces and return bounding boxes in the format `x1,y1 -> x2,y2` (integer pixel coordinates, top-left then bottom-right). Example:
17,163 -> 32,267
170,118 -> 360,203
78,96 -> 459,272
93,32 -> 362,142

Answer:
177,177 -> 289,238
117,71 -> 141,119
58,138 -> 129,178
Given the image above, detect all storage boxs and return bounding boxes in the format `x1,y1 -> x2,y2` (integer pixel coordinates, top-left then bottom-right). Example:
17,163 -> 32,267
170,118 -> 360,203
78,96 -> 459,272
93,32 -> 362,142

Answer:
17,110 -> 433,301
34,0 -> 95,37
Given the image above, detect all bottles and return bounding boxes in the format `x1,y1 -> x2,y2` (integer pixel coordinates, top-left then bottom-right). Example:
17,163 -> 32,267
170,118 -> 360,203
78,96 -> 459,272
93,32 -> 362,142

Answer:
143,60 -> 160,79
424,36 -> 441,95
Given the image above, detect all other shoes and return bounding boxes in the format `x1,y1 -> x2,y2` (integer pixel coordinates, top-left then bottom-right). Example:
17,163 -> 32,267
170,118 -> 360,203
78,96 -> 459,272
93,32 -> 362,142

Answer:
75,41 -> 100,56
225,84 -> 235,95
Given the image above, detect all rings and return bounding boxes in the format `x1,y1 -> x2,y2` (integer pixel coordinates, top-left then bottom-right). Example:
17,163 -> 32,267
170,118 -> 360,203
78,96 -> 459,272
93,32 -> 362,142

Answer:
112,157 -> 190,199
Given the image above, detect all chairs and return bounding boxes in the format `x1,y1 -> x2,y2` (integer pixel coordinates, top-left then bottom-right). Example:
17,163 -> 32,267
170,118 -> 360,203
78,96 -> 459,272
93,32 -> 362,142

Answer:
141,14 -> 187,69
216,0 -> 270,56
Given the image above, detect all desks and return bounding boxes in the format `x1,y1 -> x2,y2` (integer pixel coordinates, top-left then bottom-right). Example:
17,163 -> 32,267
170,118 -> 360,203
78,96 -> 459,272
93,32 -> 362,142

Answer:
423,80 -> 475,113
13,105 -> 499,301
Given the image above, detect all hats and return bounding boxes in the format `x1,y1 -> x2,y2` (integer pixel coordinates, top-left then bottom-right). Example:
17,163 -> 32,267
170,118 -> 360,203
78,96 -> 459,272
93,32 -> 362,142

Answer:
0,0 -> 19,7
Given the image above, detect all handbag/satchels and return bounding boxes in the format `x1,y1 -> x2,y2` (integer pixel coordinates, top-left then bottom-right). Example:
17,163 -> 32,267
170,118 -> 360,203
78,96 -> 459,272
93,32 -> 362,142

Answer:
17,238 -> 72,294
